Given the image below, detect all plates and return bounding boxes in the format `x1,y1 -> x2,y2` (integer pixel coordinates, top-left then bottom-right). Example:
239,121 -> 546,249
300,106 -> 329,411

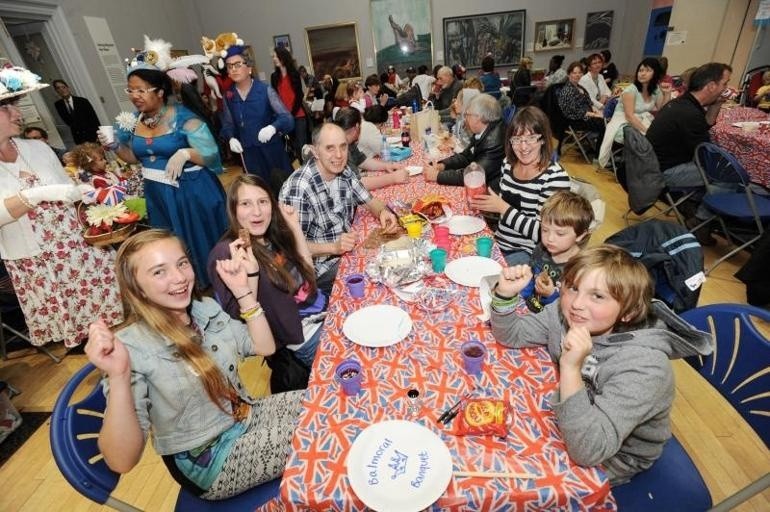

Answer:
405,166 -> 425,178
444,256 -> 504,289
345,420 -> 453,512
342,304 -> 413,350
386,136 -> 402,144
440,214 -> 487,236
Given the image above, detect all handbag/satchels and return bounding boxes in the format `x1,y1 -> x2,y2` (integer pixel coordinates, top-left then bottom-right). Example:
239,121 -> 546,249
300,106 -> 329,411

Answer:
410,101 -> 440,139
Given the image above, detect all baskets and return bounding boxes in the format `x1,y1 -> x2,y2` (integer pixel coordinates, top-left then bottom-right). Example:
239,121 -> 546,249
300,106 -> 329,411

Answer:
76,188 -> 137,247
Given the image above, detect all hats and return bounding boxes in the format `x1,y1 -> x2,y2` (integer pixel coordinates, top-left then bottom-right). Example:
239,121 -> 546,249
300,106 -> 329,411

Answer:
0,66 -> 51,99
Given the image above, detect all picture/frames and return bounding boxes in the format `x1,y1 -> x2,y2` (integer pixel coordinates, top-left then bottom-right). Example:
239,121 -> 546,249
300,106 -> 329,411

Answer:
272,34 -> 292,63
442,8 -> 527,69
368,0 -> 433,79
304,22 -> 362,81
533,19 -> 575,51
583,11 -> 613,49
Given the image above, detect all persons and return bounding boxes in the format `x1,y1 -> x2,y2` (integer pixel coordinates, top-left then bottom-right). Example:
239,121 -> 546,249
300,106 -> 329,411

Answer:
488,244 -> 712,488
0,28 -> 769,355
83,226 -> 309,502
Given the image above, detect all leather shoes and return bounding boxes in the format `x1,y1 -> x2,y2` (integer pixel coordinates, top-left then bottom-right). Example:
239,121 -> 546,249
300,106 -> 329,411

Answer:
687,216 -> 718,247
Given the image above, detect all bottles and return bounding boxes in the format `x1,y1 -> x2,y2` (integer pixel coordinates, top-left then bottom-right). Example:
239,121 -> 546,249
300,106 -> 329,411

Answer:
401,127 -> 411,148
392,110 -> 400,129
407,390 -> 421,416
412,100 -> 418,114
380,136 -> 391,162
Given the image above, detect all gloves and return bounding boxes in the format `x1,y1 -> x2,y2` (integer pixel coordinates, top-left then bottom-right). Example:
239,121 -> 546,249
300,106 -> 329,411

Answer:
257,124 -> 276,144
229,138 -> 243,153
164,147 -> 191,179
22,183 -> 75,204
95,128 -> 117,148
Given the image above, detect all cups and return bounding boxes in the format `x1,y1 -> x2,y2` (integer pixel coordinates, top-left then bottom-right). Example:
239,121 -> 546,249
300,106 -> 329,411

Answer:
477,237 -> 493,259
463,172 -> 486,212
98,124 -> 114,144
432,228 -> 452,255
428,249 -> 448,274
344,274 -> 367,298
397,105 -> 413,119
335,359 -> 361,396
459,340 -> 487,375
405,222 -> 424,239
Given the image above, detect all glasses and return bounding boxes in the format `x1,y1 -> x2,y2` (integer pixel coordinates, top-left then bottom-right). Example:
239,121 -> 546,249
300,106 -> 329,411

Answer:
124,87 -> 158,95
463,113 -> 475,118
354,80 -> 360,88
25,135 -> 45,140
226,62 -> 246,69
509,135 -> 542,146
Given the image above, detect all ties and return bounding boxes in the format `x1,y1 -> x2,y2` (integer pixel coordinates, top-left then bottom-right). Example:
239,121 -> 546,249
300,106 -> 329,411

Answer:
63,96 -> 72,111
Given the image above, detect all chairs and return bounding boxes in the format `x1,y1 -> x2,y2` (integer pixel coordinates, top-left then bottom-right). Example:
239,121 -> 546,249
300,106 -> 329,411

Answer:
695,142 -> 770,275
605,217 -> 705,312
512,82 -> 696,229
50,355 -> 281,512
612,303 -> 769,512
